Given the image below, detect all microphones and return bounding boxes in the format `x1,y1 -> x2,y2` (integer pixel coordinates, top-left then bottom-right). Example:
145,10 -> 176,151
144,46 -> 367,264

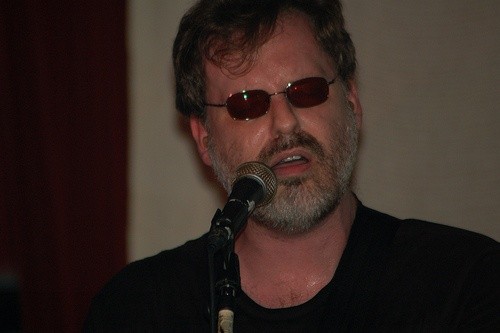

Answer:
208,160 -> 276,250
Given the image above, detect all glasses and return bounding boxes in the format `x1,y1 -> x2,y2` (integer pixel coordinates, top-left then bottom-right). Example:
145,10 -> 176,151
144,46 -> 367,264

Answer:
205,71 -> 341,121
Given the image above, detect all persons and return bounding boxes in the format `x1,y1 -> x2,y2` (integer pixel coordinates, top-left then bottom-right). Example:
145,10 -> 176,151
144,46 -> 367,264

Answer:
78,0 -> 500,333
